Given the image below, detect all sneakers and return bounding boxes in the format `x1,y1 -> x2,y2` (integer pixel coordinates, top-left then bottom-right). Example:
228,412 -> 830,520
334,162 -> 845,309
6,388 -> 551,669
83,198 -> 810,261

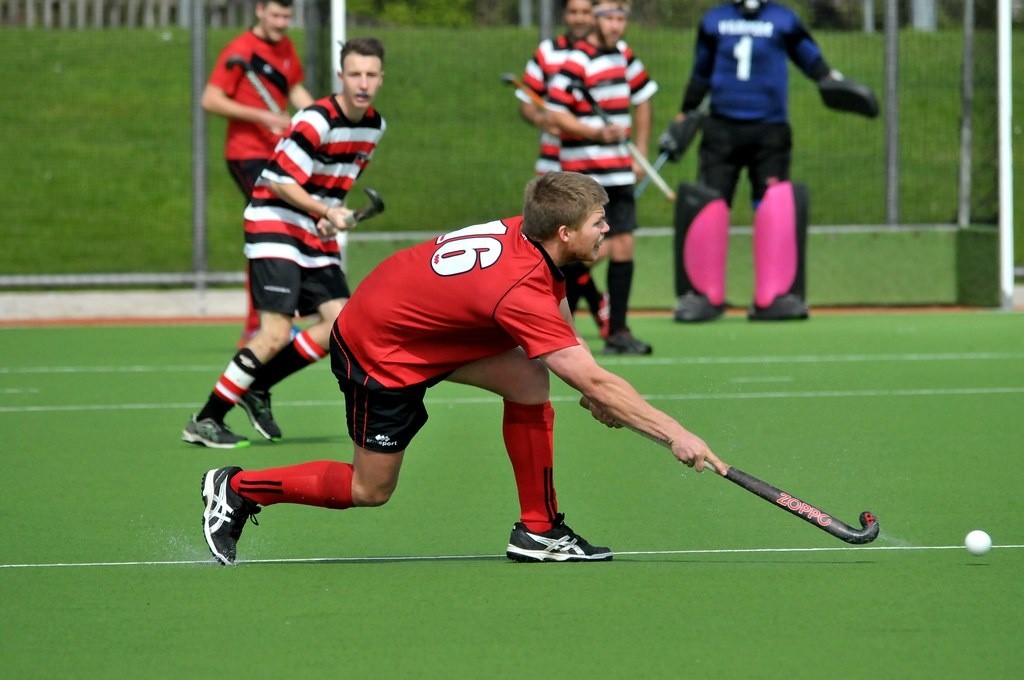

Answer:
596,292 -> 610,339
202,468 -> 262,567
507,512 -> 612,563
603,329 -> 651,356
181,413 -> 249,450
237,388 -> 282,443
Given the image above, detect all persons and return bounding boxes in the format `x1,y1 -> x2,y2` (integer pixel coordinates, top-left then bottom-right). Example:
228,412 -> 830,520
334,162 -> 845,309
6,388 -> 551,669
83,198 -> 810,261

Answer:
183,0 -> 389,451
516,0 -> 881,358
201,171 -> 729,566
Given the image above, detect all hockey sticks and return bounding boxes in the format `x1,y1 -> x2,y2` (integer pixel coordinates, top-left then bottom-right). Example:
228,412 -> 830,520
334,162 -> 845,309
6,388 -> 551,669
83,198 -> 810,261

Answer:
222,52 -> 284,118
495,70 -> 562,118
575,389 -> 883,547
631,151 -> 674,211
562,75 -> 681,205
313,182 -> 388,237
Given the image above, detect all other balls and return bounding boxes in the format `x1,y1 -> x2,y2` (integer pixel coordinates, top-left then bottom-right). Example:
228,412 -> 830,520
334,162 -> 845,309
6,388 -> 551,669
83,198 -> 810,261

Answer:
962,526 -> 996,559
